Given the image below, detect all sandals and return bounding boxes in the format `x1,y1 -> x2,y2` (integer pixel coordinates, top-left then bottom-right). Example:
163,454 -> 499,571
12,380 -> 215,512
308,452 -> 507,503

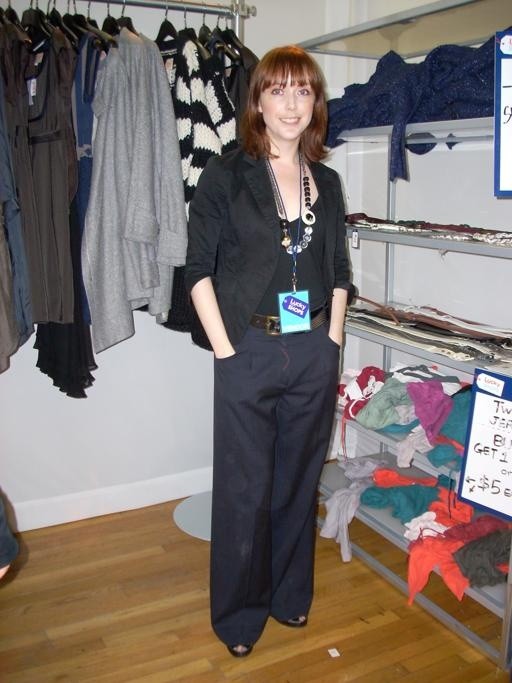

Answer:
226,643 -> 253,658
272,612 -> 308,628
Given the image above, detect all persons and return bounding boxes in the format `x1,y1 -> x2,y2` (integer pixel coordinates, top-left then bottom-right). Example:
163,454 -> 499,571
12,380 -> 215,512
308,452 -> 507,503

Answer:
179,43 -> 350,658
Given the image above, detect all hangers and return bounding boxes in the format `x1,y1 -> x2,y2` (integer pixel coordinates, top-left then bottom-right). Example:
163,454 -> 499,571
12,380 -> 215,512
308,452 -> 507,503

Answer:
0,0 -> 245,61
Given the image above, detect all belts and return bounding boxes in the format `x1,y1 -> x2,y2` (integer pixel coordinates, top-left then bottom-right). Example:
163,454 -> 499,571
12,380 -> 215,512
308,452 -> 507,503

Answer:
341,282 -> 511,371
251,309 -> 328,336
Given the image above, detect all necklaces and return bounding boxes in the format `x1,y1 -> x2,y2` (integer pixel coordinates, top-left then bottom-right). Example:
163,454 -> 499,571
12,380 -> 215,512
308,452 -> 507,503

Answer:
262,142 -> 316,256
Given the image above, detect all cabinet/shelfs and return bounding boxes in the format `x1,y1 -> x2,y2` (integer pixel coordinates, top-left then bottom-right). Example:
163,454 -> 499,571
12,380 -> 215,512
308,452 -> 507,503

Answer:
295,1 -> 512,683
